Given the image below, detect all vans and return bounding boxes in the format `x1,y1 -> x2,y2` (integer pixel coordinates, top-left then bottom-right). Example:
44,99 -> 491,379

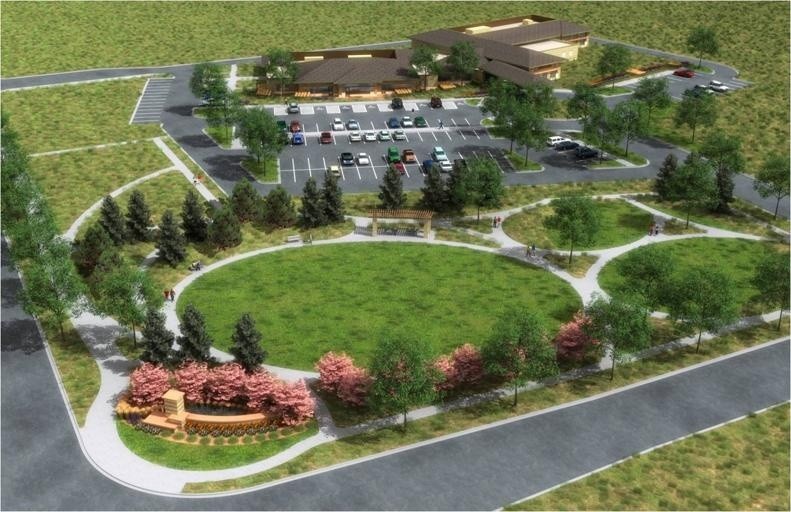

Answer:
548,135 -> 572,146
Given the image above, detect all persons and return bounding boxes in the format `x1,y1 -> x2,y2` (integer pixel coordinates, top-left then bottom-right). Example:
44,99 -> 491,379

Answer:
497,216 -> 503,229
649,222 -> 656,236
192,261 -> 201,271
163,287 -> 170,298
494,216 -> 497,228
193,174 -> 198,186
656,224 -> 659,234
170,287 -> 176,301
525,242 -> 536,258
198,174 -> 202,184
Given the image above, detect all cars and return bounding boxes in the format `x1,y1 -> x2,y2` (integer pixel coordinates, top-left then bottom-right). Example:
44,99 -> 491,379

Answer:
710,80 -> 728,92
576,147 -> 599,159
557,140 -> 579,150
319,96 -> 453,182
695,84 -> 714,96
288,102 -> 299,113
277,120 -> 304,146
674,68 -> 695,79
